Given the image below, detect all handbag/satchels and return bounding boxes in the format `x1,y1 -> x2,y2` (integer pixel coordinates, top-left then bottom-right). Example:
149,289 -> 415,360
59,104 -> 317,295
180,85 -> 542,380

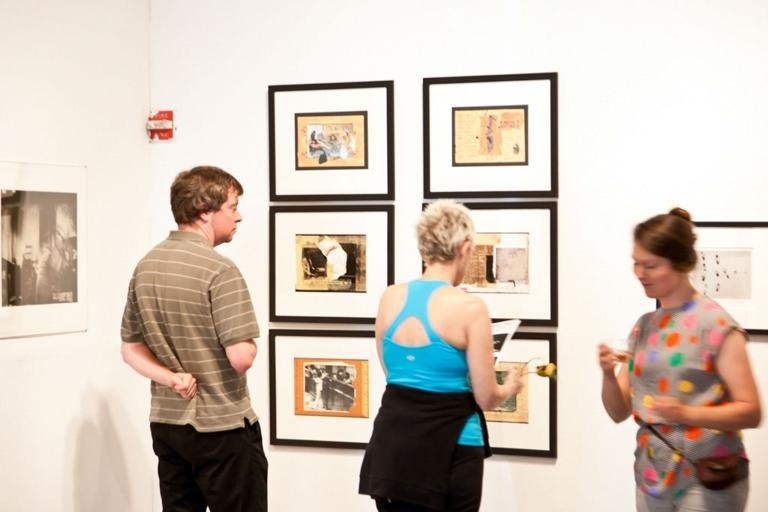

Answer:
695,454 -> 749,490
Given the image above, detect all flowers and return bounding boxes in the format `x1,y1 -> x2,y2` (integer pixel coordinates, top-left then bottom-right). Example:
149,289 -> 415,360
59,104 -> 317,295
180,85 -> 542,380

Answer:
517,355 -> 557,381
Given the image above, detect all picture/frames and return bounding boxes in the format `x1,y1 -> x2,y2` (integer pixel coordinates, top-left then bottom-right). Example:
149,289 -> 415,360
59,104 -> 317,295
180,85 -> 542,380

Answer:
1,160 -> 90,340
267,80 -> 397,203
421,71 -> 559,200
421,200 -> 558,327
268,203 -> 397,325
656,220 -> 768,336
482,332 -> 559,458
268,327 -> 387,450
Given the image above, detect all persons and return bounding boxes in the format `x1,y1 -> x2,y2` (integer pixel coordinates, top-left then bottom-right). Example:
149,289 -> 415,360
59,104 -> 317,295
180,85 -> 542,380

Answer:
308,128 -> 354,164
304,364 -> 353,410
19,203 -> 77,303
120,165 -> 266,512
598,208 -> 761,512
315,235 -> 347,279
357,198 -> 525,512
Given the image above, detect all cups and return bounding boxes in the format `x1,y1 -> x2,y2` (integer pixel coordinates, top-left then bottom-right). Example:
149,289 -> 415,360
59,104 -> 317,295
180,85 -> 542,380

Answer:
607,338 -> 636,363
